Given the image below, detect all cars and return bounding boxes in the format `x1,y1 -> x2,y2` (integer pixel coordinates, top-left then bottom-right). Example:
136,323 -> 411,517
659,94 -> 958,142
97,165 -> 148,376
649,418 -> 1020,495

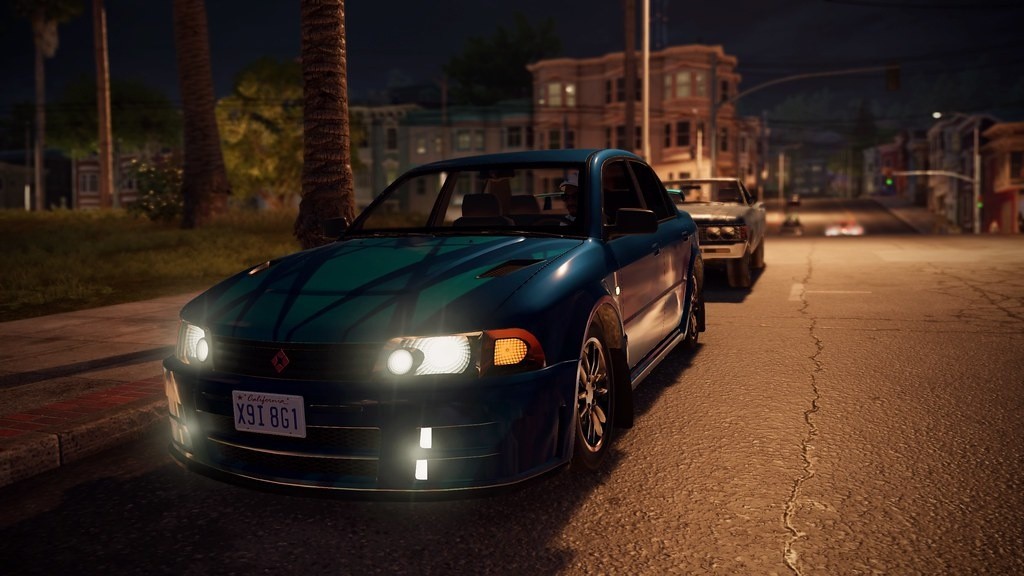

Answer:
162,147 -> 704,502
654,177 -> 767,291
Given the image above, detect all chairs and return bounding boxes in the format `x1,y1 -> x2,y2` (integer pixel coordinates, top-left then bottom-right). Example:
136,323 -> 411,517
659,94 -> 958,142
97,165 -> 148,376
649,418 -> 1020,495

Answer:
666,189 -> 685,203
719,188 -> 739,200
452,194 -> 517,232
